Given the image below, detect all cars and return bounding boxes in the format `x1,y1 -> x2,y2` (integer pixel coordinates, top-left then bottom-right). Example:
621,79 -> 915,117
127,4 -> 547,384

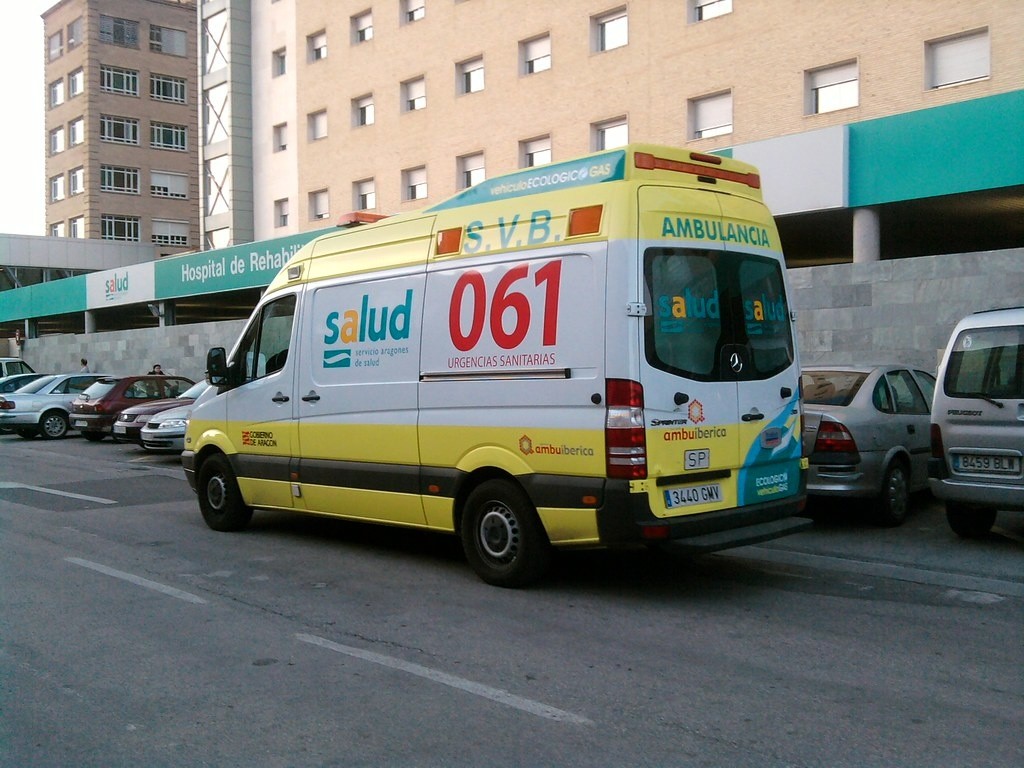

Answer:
68,374 -> 198,441
0,373 -> 149,441
790,364 -> 937,532
113,379 -> 214,441
1,373 -> 85,393
139,403 -> 190,452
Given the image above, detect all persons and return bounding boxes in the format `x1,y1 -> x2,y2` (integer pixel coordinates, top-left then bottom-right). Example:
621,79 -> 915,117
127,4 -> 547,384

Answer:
145,364 -> 175,397
80,359 -> 89,373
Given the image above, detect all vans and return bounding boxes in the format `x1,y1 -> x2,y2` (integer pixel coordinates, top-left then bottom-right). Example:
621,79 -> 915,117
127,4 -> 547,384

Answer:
926,305 -> 1024,537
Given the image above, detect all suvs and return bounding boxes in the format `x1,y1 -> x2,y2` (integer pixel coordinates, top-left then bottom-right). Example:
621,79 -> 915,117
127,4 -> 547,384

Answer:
0,357 -> 37,379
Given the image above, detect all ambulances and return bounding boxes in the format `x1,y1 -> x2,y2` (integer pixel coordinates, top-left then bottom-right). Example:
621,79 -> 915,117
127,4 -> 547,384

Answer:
180,150 -> 809,587
